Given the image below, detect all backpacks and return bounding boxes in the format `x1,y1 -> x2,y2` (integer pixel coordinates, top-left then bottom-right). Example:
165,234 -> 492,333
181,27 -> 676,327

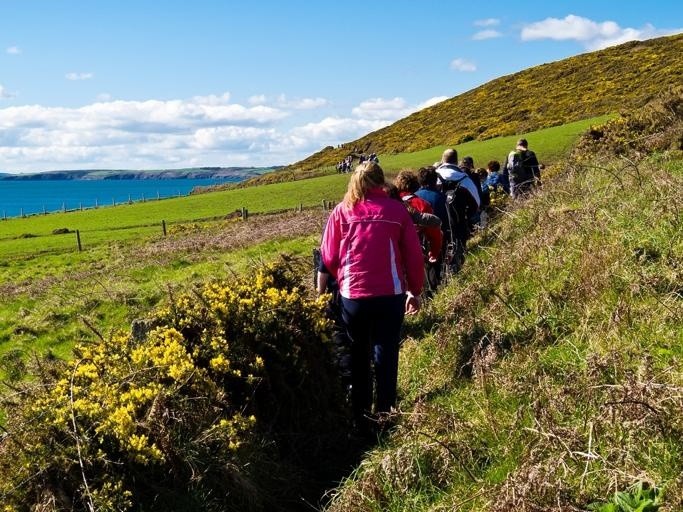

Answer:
436,173 -> 468,226
514,149 -> 534,183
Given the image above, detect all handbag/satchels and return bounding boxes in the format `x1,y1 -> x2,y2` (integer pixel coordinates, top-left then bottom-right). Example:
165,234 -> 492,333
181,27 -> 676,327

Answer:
445,240 -> 463,266
